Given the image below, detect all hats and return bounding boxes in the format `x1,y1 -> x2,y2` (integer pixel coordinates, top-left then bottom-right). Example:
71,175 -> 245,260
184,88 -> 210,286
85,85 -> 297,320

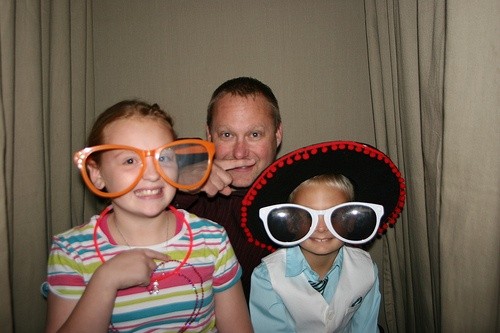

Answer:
237,139 -> 406,253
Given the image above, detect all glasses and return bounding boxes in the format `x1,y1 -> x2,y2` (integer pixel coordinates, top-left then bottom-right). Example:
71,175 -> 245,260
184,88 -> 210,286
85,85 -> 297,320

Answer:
259,201 -> 384,247
74,138 -> 215,197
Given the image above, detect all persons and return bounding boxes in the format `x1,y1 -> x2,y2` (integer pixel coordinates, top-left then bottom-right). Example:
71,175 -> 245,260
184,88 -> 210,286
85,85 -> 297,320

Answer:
239,140 -> 406,333
171,77 -> 283,303
39,99 -> 254,333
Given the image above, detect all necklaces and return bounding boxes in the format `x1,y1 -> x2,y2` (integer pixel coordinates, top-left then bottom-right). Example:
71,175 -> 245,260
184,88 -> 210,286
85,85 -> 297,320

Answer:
93,205 -> 194,282
112,210 -> 169,295
